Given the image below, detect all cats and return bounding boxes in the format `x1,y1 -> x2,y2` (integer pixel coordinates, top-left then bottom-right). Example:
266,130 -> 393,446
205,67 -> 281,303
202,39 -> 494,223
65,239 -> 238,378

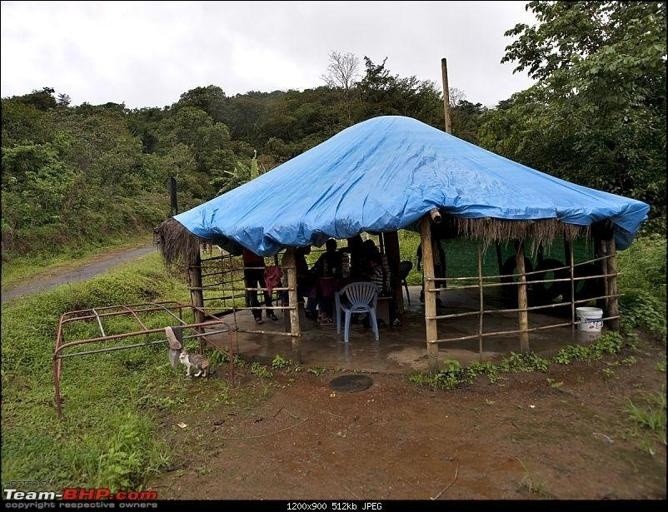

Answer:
179,350 -> 210,377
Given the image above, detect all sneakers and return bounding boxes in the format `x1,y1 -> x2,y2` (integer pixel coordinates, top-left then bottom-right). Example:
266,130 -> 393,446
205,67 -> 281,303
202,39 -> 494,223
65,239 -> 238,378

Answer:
436,298 -> 442,304
255,317 -> 264,324
420,290 -> 425,303
265,312 -> 277,321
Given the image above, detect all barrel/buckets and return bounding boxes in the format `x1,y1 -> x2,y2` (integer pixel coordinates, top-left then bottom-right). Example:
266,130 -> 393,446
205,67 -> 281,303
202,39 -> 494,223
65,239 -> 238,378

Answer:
575,307 -> 604,333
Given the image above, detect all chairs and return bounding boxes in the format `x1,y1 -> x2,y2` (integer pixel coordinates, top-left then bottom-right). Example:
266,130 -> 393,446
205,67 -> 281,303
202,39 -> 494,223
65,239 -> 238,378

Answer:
333,282 -> 381,342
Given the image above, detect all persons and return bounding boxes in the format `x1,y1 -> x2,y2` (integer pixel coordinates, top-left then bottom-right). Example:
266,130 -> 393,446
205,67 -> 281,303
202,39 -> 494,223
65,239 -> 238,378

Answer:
243,246 -> 278,324
281,235 -> 401,326
417,238 -> 446,303
